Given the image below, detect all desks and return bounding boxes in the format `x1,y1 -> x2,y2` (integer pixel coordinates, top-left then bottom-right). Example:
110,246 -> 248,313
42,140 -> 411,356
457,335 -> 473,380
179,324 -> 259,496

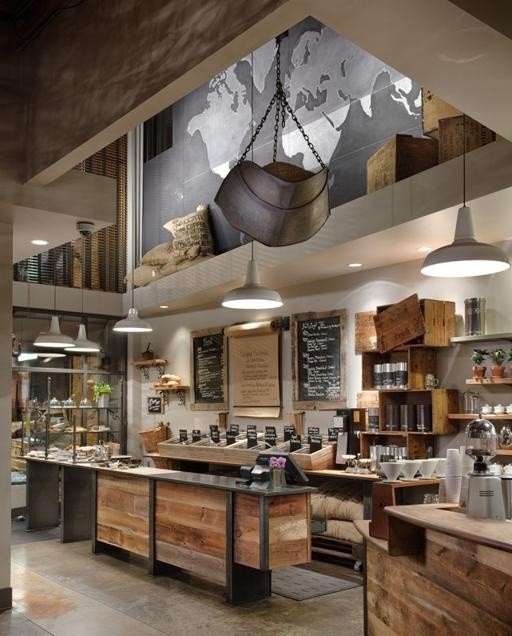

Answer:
16,455 -> 319,608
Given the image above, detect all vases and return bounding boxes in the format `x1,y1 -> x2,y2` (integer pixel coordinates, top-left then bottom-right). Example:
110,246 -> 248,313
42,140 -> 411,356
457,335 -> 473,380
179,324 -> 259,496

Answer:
270,468 -> 285,489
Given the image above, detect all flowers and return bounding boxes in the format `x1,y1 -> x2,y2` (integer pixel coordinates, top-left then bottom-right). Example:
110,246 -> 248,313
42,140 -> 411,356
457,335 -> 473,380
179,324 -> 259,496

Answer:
270,456 -> 286,470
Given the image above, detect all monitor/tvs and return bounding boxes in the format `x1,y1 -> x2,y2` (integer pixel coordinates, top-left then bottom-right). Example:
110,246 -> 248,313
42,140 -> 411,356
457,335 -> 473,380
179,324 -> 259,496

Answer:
256,452 -> 310,482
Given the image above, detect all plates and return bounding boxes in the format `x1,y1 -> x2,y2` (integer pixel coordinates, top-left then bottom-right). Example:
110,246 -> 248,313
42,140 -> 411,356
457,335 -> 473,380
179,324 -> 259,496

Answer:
382,473 -> 447,483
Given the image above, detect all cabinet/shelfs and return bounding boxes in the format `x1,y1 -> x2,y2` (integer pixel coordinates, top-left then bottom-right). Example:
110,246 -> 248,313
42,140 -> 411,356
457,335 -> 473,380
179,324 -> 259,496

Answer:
131,359 -> 189,405
359,294 -> 461,461
44,376 -> 123,460
450,332 -> 512,459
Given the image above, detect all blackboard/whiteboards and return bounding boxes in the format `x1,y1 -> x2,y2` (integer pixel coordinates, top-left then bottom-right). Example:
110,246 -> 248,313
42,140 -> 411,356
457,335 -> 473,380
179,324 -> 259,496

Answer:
146,394 -> 165,415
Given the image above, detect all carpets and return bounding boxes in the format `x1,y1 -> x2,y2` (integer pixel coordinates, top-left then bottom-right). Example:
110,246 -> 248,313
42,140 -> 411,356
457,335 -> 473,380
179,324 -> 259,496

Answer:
271,564 -> 363,602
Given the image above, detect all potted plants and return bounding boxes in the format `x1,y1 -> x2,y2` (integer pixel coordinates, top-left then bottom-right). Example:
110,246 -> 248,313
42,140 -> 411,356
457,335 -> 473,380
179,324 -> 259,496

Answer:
471,348 -> 490,378
490,349 -> 506,378
94,380 -> 111,408
506,349 -> 512,378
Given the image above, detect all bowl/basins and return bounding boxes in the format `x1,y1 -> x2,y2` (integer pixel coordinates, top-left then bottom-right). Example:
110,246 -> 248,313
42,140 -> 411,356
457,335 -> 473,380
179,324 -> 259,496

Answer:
428,457 -> 448,475
397,460 -> 420,479
378,461 -> 403,481
415,459 -> 438,478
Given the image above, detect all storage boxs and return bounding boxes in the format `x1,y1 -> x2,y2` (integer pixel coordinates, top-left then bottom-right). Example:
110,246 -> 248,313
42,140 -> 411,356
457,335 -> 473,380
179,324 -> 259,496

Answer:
438,115 -> 496,164
367,134 -> 439,194
421,87 -> 465,141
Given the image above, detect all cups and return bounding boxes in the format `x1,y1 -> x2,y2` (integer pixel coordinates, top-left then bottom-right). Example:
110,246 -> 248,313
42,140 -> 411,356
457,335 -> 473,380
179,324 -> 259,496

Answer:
493,405 -> 505,414
424,373 -> 438,390
445,446 -> 473,503
505,404 -> 511,416
489,463 -> 512,477
480,404 -> 492,414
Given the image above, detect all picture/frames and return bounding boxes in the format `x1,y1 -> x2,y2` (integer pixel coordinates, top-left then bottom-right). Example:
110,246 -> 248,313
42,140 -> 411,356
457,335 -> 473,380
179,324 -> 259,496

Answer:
146,396 -> 164,415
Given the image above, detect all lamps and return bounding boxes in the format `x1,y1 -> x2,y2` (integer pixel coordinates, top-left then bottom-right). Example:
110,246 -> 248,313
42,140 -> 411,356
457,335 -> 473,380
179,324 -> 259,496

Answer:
221,52 -> 284,310
33,246 -> 76,348
420,113 -> 511,279
64,221 -> 101,354
112,127 -> 154,333
